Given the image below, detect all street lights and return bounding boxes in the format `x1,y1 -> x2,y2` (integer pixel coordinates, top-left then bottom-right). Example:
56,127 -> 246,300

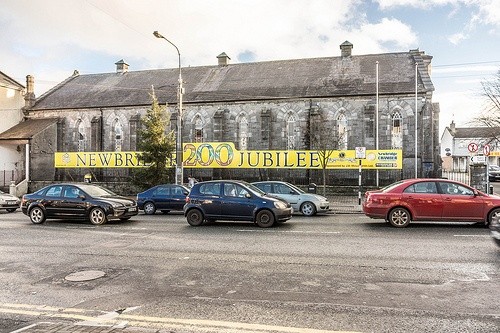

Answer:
153,30 -> 184,185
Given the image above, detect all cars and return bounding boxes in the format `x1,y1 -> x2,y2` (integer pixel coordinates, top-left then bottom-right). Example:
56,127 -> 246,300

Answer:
0,190 -> 21,214
486,164 -> 500,182
136,184 -> 189,215
361,178 -> 500,232
238,180 -> 331,217
20,182 -> 139,225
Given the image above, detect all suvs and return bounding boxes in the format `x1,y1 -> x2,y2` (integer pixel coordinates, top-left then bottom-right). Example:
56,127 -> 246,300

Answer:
183,180 -> 295,228
